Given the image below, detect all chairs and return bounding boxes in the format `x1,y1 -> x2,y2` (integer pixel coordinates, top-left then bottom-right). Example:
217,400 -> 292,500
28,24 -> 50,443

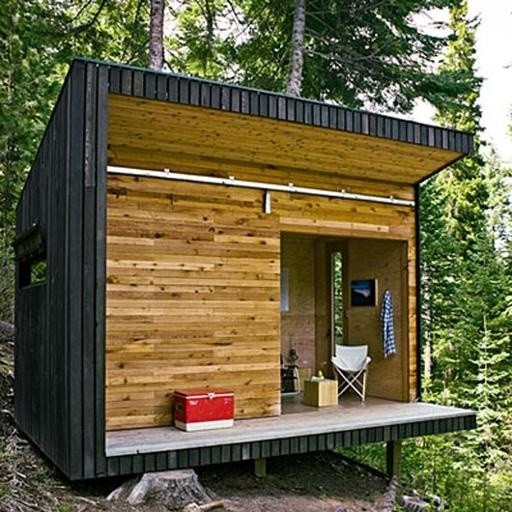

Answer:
281,354 -> 301,395
330,343 -> 372,402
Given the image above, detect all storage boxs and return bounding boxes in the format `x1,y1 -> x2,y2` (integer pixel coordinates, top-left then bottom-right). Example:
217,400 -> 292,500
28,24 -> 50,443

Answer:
302,378 -> 339,406
173,388 -> 234,432
